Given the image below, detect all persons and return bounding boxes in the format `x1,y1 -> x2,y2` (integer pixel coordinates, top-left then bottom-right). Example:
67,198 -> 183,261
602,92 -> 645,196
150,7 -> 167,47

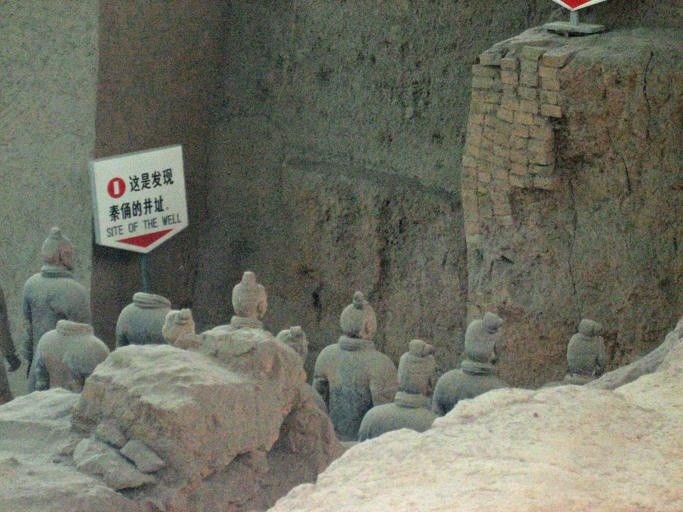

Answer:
21,228 -> 90,360
203,271 -> 267,333
0,287 -> 21,405
432,312 -> 510,417
540,318 -> 605,388
162,308 -> 195,348
28,319 -> 110,394
115,292 -> 172,350
357,339 -> 443,442
312,291 -> 397,442
276,326 -> 326,412
